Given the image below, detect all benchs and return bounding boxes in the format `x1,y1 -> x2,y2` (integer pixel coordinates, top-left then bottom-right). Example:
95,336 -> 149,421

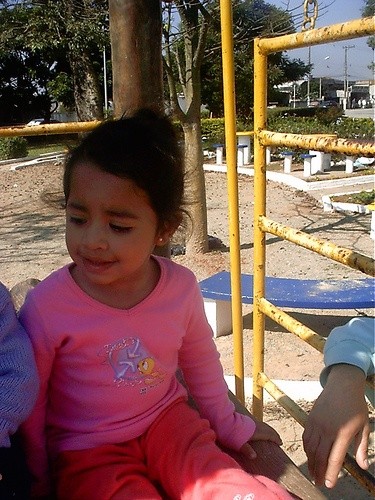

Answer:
199,272 -> 375,339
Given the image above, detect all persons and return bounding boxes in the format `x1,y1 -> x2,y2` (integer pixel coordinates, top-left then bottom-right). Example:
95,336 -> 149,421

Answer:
21,106 -> 298,500
302,317 -> 375,488
0,281 -> 40,500
